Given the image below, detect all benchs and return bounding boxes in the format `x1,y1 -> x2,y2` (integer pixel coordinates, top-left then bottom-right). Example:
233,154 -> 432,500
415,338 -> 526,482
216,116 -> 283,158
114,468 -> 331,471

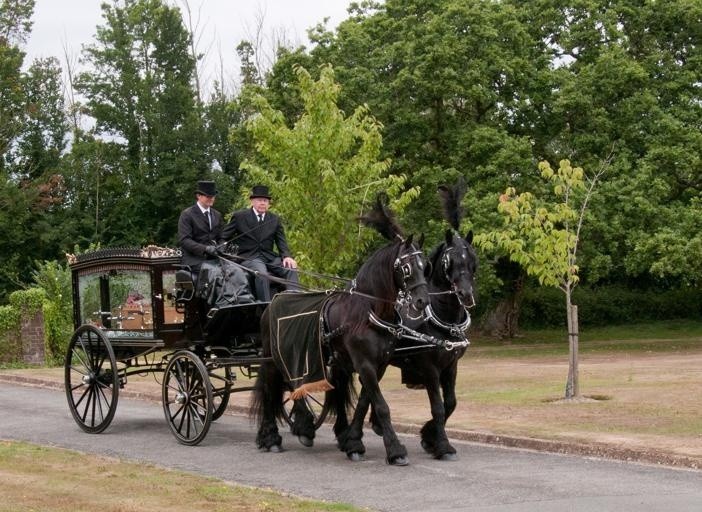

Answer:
171,258 -> 286,337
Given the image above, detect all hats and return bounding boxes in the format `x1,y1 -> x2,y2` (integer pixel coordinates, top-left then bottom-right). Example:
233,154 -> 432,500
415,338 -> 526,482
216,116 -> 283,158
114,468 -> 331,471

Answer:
193,180 -> 219,197
249,185 -> 273,200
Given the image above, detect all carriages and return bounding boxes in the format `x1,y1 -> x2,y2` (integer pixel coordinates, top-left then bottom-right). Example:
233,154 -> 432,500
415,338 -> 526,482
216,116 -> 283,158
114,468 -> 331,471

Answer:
64,182 -> 477,467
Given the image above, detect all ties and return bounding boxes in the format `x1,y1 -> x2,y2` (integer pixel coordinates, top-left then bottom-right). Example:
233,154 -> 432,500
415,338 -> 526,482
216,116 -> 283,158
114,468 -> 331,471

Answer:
258,214 -> 264,231
204,211 -> 210,229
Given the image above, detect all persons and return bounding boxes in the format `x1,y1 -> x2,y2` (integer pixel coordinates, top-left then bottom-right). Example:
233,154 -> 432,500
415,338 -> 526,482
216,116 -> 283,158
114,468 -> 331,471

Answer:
220,186 -> 300,300
178,180 -> 252,303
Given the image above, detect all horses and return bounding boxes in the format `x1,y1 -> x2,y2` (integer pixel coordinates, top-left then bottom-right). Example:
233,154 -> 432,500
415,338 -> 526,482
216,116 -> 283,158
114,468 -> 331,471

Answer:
325,229 -> 479,463
248,229 -> 430,466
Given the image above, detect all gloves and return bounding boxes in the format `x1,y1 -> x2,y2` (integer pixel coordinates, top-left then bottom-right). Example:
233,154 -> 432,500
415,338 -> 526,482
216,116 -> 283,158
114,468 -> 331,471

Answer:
206,239 -> 228,257
